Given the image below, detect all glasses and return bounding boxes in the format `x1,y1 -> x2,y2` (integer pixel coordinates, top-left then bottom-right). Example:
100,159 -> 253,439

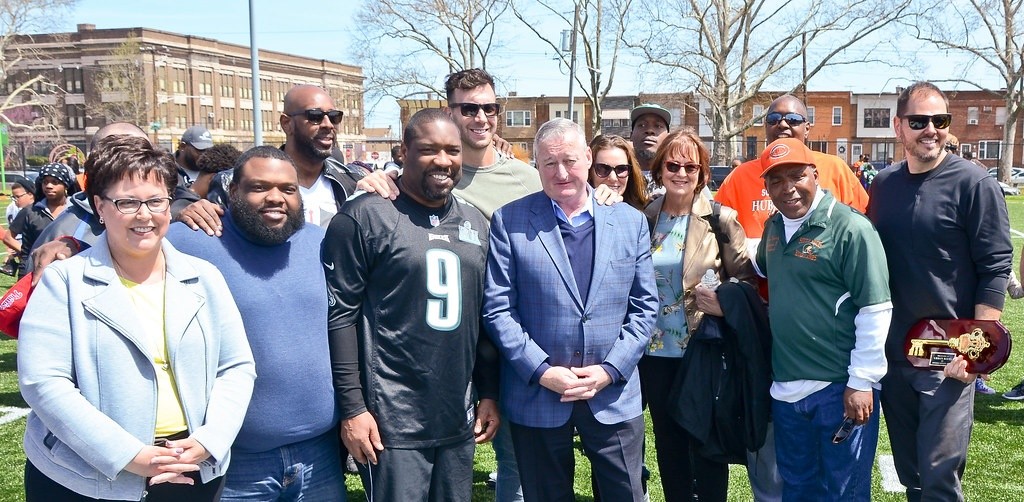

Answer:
592,163 -> 633,178
831,415 -> 857,444
448,102 -> 500,117
286,108 -> 344,125
662,161 -> 703,175
901,112 -> 953,130
103,195 -> 172,215
765,111 -> 807,127
10,191 -> 29,201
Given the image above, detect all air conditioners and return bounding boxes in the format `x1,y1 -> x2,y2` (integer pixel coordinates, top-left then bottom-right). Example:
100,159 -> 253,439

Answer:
967,119 -> 978,126
207,113 -> 214,119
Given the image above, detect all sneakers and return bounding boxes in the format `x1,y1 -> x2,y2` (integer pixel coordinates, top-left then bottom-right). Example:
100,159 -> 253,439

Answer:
346,454 -> 358,474
1002,383 -> 1024,400
974,377 -> 996,395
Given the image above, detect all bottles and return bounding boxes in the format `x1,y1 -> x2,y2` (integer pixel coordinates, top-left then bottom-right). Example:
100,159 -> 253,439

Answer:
700,268 -> 720,292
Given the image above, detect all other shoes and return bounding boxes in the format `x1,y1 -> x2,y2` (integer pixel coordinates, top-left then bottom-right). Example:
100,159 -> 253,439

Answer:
641,479 -> 650,502
487,469 -> 499,489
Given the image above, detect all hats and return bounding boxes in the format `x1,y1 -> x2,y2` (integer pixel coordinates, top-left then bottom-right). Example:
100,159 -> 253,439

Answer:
630,103 -> 672,126
17,176 -> 36,196
760,138 -> 816,178
182,126 -> 215,151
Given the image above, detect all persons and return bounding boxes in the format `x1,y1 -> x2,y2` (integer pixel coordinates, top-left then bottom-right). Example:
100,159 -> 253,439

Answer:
745,139 -> 895,501
16,136 -> 260,500
477,118 -> 659,502
0,67 -> 1023,502
319,107 -> 501,501
860,83 -> 1014,501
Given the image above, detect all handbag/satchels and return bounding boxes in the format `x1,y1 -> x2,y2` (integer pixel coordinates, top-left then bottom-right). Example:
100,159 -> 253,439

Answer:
693,311 -> 726,344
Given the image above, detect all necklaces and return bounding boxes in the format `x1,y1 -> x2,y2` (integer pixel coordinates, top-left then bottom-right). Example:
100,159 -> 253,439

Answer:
427,214 -> 441,228
112,252 -> 170,371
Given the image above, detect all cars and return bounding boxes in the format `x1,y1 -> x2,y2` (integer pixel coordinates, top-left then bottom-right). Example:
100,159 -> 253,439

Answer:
987,166 -> 1024,184
997,181 -> 1021,196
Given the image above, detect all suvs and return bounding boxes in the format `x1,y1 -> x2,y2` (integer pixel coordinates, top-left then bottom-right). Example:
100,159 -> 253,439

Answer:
0,170 -> 39,195
708,166 -> 734,187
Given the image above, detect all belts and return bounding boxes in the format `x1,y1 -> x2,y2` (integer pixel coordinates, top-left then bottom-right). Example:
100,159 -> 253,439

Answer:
154,433 -> 188,447
